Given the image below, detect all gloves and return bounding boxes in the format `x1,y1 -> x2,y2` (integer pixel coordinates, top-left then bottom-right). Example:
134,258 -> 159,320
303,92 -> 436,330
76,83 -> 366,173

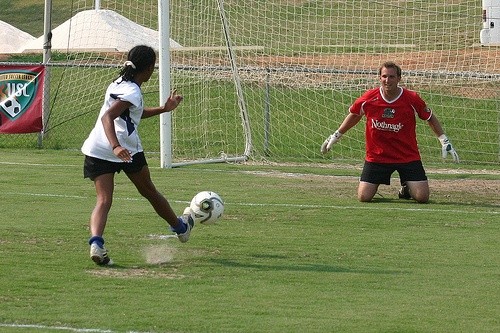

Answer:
320,130 -> 343,155
438,134 -> 459,163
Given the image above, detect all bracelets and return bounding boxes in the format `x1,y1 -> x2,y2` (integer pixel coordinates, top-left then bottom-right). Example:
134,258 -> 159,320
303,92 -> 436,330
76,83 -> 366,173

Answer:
112,145 -> 121,150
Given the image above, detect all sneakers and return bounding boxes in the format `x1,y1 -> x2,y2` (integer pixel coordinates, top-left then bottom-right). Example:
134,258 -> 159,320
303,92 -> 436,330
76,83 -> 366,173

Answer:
397,186 -> 411,199
89,243 -> 114,266
177,206 -> 195,243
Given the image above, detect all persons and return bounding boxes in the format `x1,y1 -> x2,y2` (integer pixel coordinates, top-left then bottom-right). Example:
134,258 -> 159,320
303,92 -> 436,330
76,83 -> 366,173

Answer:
321,62 -> 459,203
81,46 -> 196,266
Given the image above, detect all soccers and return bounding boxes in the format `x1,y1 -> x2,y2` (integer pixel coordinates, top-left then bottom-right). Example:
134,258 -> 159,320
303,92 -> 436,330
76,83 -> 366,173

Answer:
189,188 -> 226,226
